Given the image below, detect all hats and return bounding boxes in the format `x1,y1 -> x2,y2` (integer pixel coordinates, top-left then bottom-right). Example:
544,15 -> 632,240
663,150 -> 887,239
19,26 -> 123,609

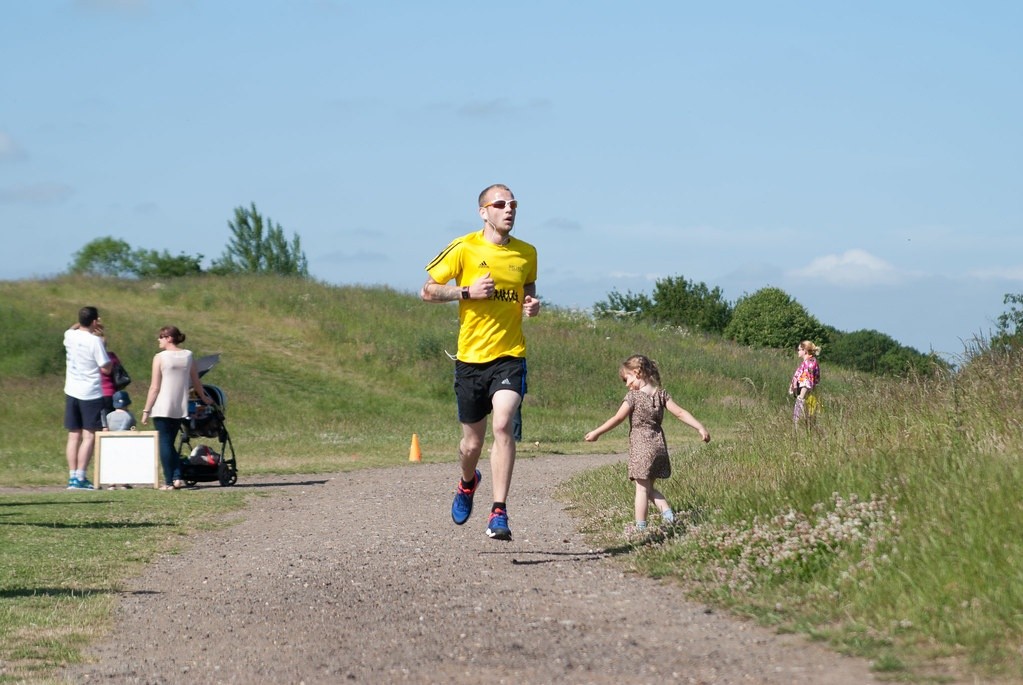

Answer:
111,391 -> 132,408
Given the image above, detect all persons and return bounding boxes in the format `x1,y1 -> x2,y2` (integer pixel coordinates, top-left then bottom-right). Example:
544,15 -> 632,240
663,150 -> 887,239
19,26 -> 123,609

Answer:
583,354 -> 711,530
141,324 -> 214,492
419,182 -> 540,540
61,306 -> 136,490
787,339 -> 821,438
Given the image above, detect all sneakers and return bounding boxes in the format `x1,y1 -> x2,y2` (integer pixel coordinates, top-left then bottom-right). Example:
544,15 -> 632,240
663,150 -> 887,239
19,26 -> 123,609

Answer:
67,478 -> 95,491
451,469 -> 481,527
486,510 -> 513,540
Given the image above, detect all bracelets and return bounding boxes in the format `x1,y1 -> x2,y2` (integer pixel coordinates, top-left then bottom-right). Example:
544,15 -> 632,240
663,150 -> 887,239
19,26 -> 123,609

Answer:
142,409 -> 150,413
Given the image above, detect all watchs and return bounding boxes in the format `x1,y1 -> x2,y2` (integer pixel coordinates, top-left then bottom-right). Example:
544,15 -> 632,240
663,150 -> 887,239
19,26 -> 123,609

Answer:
461,285 -> 469,299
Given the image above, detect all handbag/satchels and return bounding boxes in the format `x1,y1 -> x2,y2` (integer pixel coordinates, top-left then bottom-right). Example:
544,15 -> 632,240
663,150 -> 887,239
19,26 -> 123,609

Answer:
109,364 -> 131,390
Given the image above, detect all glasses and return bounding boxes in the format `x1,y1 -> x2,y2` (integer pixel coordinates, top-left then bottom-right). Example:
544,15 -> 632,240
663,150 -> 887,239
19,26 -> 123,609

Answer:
483,199 -> 519,210
158,335 -> 171,339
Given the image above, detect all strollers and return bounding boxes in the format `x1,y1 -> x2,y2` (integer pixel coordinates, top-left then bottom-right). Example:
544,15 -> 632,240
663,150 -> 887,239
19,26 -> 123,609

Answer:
166,384 -> 237,488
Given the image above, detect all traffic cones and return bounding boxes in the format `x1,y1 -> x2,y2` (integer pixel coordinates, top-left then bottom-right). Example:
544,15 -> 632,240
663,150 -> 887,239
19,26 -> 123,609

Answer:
409,434 -> 423,464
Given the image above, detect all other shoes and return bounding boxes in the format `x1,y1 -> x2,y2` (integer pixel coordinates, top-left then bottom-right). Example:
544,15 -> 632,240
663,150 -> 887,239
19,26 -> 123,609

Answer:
633,510 -> 676,532
161,478 -> 190,490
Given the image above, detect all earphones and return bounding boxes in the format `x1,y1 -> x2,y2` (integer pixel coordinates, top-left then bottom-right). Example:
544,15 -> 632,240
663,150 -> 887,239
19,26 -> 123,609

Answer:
484,213 -> 487,220
